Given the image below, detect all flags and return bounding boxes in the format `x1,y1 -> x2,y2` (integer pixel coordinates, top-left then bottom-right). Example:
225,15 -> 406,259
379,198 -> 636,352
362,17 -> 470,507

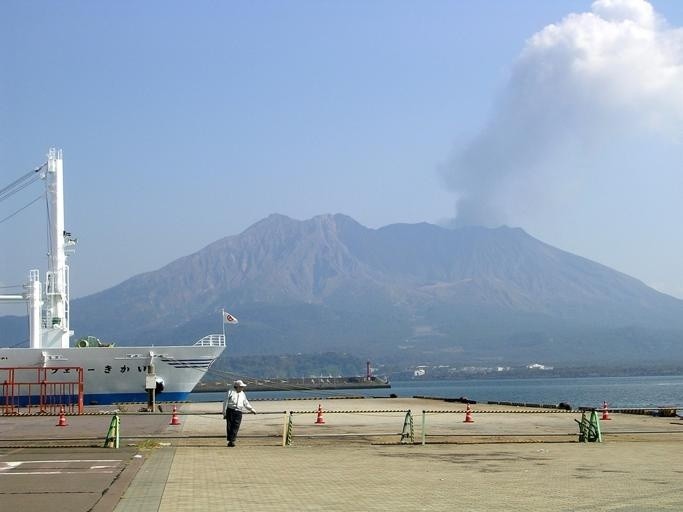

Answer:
224,312 -> 239,324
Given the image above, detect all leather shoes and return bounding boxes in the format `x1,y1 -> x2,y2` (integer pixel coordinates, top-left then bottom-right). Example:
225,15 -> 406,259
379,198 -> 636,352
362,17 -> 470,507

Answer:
228,441 -> 236,447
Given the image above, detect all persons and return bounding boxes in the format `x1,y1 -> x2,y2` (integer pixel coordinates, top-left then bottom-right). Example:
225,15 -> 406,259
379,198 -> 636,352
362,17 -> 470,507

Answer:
222,379 -> 256,447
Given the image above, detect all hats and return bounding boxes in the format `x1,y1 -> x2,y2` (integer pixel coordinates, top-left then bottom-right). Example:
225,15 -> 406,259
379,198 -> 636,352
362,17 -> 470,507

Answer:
233,379 -> 247,387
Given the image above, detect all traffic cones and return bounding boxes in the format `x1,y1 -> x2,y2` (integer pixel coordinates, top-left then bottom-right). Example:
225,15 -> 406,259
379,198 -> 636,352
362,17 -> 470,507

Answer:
463,402 -> 476,422
600,400 -> 612,420
169,404 -> 181,425
314,404 -> 326,423
59,406 -> 67,426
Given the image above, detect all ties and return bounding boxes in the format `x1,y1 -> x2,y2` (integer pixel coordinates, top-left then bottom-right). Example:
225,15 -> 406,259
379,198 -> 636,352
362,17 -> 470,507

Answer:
236,393 -> 240,408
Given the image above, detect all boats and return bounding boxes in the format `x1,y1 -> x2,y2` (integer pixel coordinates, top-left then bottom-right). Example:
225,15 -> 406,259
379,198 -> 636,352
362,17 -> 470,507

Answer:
192,361 -> 392,392
0,145 -> 227,404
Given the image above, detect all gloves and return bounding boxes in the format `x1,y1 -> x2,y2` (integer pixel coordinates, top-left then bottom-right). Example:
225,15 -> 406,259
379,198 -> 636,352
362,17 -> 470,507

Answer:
251,409 -> 256,415
